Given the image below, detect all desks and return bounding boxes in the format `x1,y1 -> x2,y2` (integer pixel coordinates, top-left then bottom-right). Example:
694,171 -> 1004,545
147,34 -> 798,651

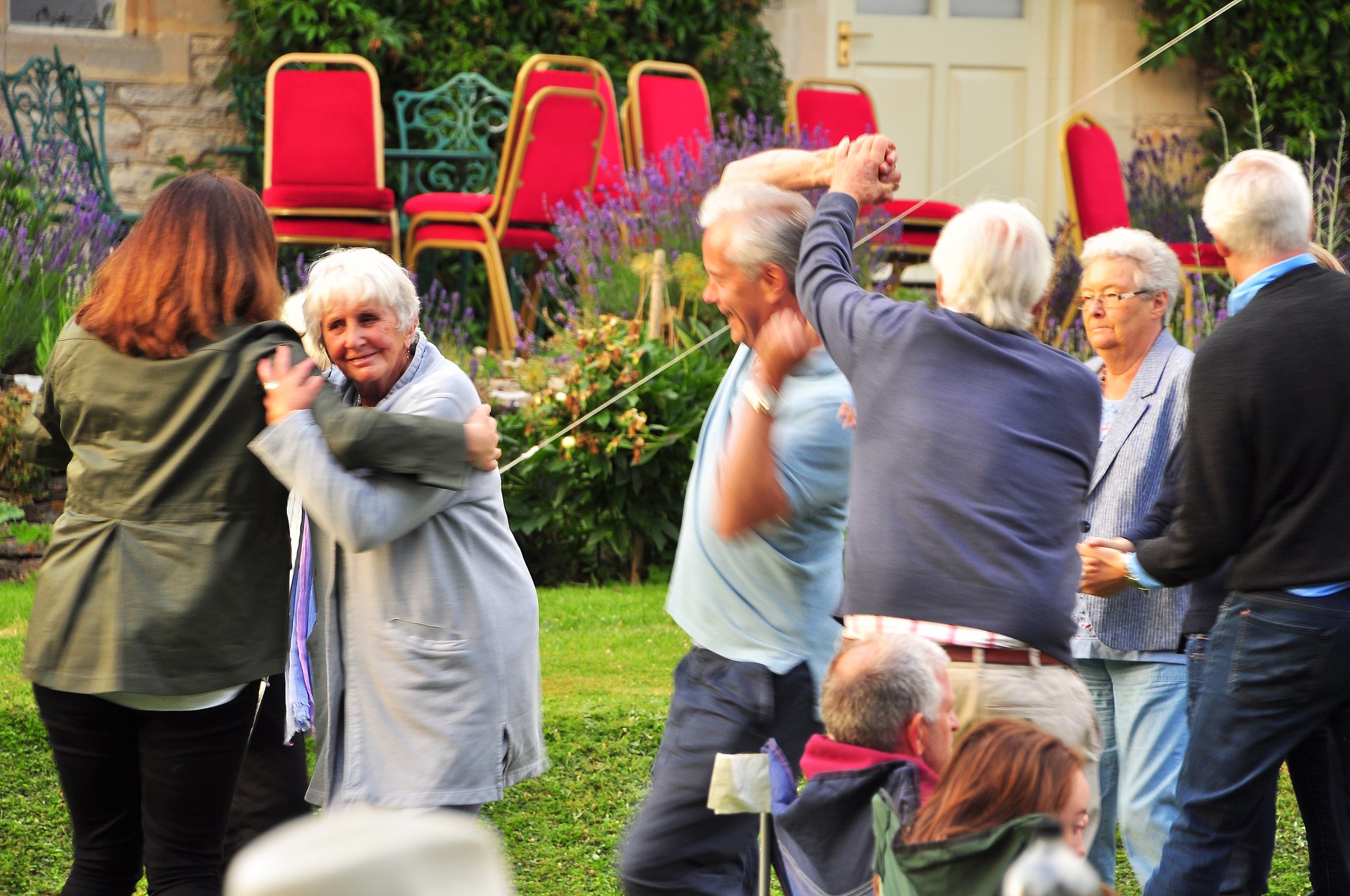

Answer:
217,145 -> 497,160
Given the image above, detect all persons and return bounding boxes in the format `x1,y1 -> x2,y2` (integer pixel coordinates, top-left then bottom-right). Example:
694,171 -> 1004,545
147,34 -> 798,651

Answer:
798,613 -> 1115,896
619,132 -> 903,896
21,170 -> 502,896
1075,149 -> 1350,896
225,248 -> 555,896
795,131 -> 1116,896
1062,228 -> 1200,896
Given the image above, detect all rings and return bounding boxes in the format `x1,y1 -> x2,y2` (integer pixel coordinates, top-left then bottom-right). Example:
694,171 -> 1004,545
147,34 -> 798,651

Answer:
264,381 -> 278,389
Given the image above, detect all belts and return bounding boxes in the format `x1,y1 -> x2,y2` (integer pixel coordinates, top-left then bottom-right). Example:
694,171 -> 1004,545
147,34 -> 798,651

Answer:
842,638 -> 1062,666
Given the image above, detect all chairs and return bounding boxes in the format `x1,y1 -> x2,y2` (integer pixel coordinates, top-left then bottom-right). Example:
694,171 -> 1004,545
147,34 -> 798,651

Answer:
0,43 -> 144,244
393,72 -> 514,231
262,52 -> 1227,360
759,738 -> 1062,896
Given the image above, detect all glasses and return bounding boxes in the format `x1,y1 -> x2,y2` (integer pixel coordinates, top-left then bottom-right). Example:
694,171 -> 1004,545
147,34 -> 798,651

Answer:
1072,289 -> 1147,311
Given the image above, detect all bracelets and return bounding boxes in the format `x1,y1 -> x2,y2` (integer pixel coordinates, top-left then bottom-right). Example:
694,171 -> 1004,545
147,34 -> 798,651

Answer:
739,372 -> 785,420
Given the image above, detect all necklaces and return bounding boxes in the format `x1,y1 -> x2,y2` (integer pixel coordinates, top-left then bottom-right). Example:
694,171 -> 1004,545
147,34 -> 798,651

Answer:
356,349 -> 412,408
